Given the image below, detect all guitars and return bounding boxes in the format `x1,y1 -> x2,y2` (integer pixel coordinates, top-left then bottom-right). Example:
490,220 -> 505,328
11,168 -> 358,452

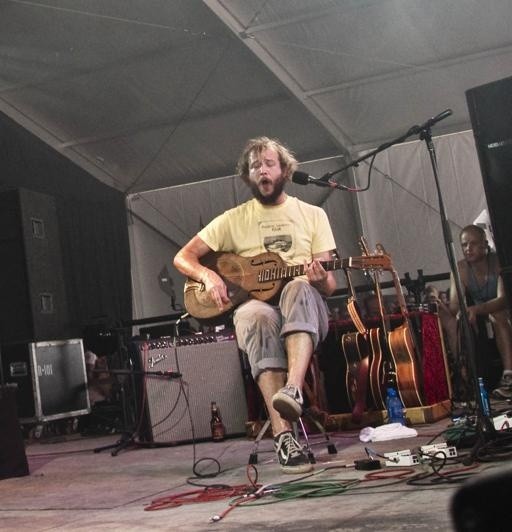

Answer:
330,236 -> 424,412
184,252 -> 393,326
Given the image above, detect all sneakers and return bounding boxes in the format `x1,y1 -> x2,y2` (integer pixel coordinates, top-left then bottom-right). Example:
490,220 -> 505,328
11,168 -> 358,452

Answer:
493,373 -> 511,399
272,384 -> 304,423
272,431 -> 313,475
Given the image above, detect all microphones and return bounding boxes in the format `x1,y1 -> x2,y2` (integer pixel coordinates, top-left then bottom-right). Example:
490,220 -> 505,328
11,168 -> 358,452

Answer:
292,171 -> 348,192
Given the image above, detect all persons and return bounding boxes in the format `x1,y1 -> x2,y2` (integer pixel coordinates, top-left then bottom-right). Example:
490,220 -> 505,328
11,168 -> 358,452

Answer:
173,135 -> 340,474
29,350 -> 117,440
424,285 -> 451,328
438,291 -> 449,304
446,224 -> 511,401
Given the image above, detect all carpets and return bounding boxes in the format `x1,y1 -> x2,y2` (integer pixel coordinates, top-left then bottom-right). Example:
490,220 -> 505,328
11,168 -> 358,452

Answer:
0,432 -> 361,509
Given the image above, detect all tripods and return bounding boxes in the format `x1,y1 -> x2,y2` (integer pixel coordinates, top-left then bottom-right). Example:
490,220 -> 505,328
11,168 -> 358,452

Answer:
93,333 -> 179,456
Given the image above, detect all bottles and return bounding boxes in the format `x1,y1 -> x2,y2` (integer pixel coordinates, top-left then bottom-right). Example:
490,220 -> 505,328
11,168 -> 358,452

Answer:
209,401 -> 226,442
478,377 -> 491,417
385,388 -> 406,425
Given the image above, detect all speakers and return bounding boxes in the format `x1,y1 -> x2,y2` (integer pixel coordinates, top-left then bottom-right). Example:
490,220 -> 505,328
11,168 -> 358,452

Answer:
465,76 -> 512,319
0,185 -> 70,344
